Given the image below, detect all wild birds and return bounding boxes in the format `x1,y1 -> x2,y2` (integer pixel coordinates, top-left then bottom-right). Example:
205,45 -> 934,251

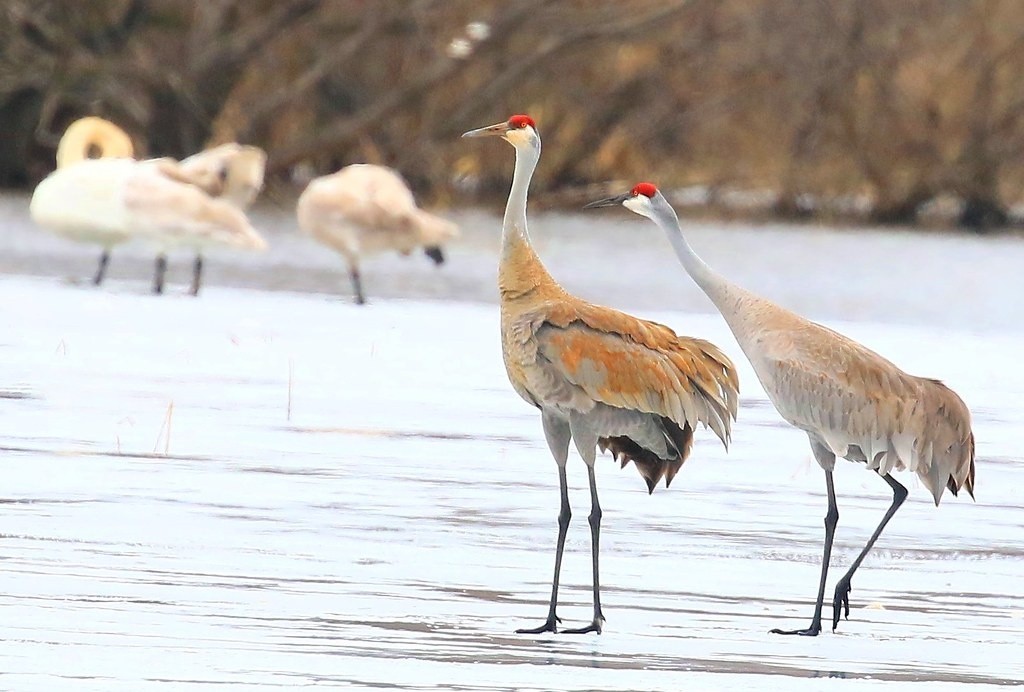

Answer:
580,185 -> 979,637
29,113 -> 267,292
460,117 -> 740,637
295,162 -> 462,305
